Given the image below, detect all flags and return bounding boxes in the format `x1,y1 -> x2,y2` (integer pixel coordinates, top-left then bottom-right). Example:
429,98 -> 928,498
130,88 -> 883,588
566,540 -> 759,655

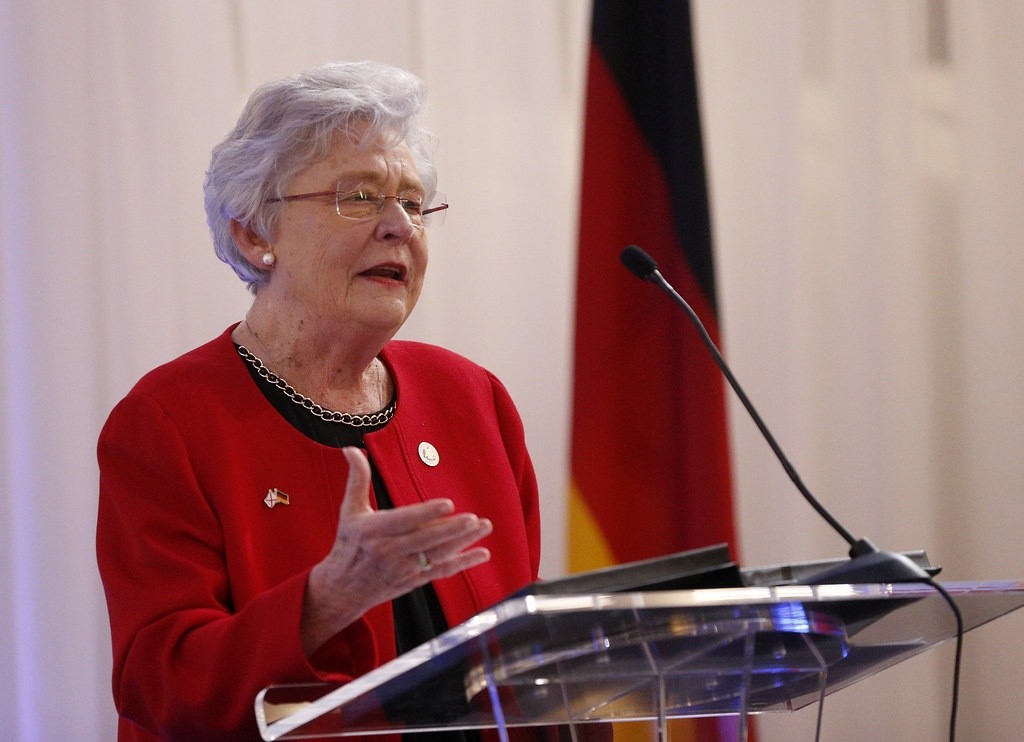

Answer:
567,1 -> 759,741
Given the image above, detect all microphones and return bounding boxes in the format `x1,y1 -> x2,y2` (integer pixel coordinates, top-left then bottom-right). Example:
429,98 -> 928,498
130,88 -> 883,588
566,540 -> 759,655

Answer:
617,245 -> 931,584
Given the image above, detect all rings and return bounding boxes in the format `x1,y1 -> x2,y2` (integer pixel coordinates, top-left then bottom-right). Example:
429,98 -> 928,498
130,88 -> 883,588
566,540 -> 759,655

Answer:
416,551 -> 433,571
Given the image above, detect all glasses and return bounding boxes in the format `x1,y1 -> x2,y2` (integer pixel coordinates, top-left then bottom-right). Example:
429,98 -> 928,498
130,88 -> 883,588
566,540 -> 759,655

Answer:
264,178 -> 449,228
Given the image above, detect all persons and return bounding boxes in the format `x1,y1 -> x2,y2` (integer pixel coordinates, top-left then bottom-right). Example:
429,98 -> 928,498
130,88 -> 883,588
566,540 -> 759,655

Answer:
95,59 -> 542,742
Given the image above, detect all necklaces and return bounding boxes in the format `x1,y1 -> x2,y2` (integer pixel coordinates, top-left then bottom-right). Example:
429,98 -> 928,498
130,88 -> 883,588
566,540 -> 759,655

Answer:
235,342 -> 397,426
